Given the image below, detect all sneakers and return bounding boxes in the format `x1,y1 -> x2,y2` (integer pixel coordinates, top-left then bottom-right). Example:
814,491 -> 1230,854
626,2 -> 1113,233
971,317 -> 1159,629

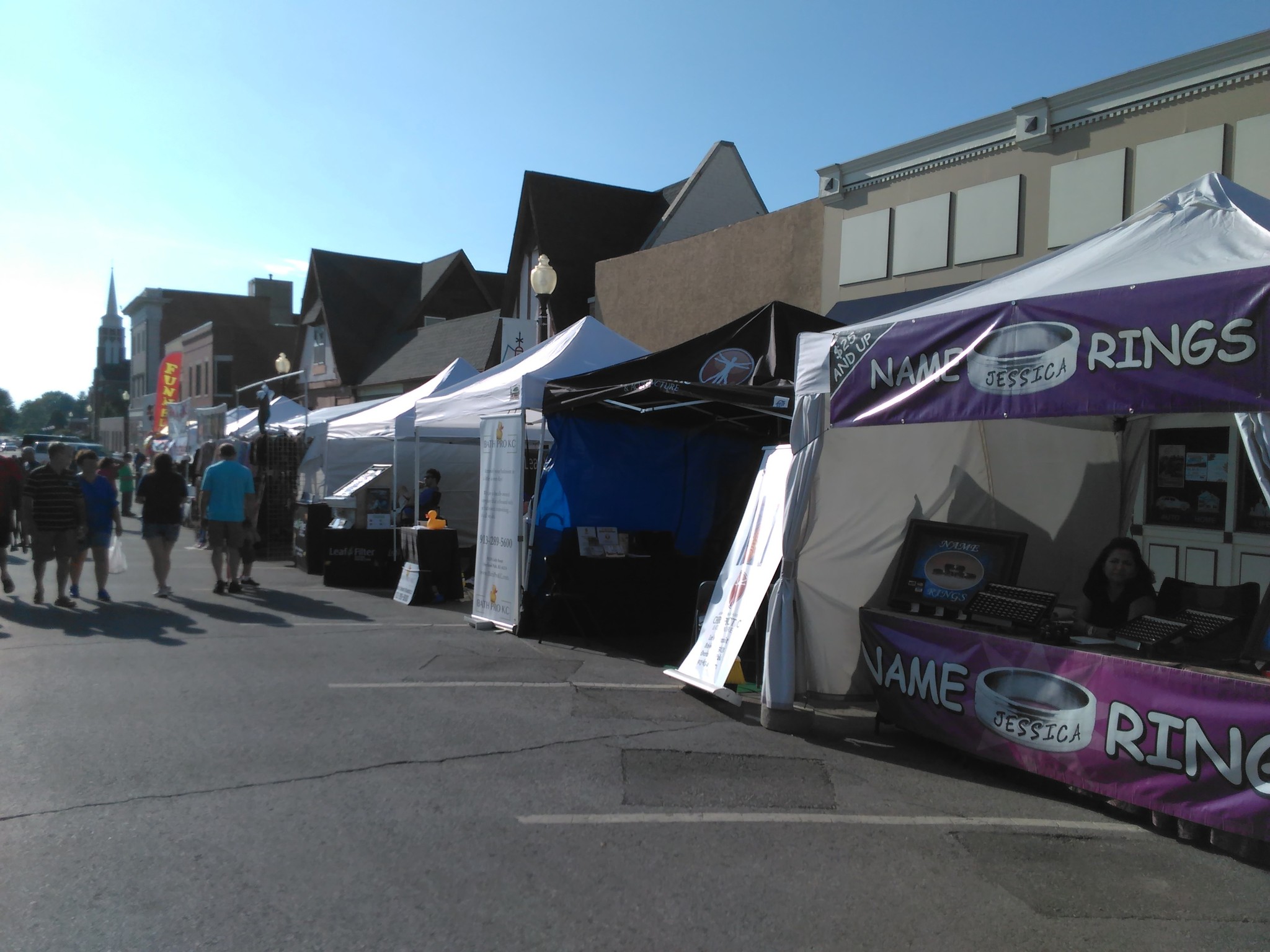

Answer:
229,583 -> 242,593
241,577 -> 260,589
213,580 -> 225,595
34,586 -> 44,602
96,589 -> 112,601
223,581 -> 232,589
154,585 -> 173,598
54,596 -> 77,608
69,586 -> 81,598
3,575 -> 15,594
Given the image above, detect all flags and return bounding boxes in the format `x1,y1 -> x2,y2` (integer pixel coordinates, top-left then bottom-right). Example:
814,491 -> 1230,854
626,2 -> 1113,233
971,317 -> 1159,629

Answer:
153,350 -> 183,427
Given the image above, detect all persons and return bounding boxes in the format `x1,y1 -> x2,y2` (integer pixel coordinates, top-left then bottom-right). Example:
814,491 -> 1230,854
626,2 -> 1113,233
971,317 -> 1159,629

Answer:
0,432 -> 145,627
1050,536 -> 1157,638
254,384 -> 275,433
224,501 -> 262,590
402,469 -> 441,525
196,443 -> 260,593
136,453 -> 191,599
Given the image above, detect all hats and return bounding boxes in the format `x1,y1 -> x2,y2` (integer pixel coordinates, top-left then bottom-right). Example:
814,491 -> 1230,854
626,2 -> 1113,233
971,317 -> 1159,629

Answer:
181,454 -> 191,460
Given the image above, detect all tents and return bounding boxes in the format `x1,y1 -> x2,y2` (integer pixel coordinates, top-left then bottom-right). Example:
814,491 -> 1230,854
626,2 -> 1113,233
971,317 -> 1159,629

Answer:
517,298 -> 846,668
155,393 -> 311,442
749,169 -> 1270,858
404,314 -> 652,634
245,390 -> 402,505
319,354 -> 480,562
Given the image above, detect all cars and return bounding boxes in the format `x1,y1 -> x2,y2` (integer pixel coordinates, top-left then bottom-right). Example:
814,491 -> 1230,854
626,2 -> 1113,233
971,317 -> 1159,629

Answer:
0,436 -> 23,452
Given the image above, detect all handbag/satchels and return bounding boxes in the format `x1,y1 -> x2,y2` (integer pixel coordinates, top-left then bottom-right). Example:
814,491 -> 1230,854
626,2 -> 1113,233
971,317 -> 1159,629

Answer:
108,536 -> 128,574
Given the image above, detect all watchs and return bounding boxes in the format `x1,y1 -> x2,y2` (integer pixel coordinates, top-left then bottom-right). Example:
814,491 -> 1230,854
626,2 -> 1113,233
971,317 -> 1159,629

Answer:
1087,625 -> 1095,635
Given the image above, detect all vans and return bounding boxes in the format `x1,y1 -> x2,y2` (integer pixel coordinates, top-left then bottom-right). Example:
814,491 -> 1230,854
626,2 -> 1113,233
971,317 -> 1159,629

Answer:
20,433 -> 107,465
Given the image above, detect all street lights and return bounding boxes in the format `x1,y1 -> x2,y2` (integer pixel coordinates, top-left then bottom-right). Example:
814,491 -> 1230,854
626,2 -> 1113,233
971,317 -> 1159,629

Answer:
87,405 -> 93,438
530,254 -> 557,344
68,412 -> 74,435
275,352 -> 291,396
122,391 -> 130,455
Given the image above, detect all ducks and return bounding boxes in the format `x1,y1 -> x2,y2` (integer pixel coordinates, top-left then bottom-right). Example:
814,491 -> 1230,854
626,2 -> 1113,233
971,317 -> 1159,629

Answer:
425,510 -> 447,530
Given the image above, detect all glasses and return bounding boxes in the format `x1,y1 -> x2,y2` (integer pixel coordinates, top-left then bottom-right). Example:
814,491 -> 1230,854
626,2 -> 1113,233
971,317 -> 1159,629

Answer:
423,475 -> 431,479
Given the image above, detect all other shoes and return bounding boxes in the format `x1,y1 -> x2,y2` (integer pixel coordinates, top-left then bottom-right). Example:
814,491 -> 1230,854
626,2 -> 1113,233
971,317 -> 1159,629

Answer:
134,499 -> 145,503
192,542 -> 215,551
11,542 -> 33,553
121,510 -> 136,517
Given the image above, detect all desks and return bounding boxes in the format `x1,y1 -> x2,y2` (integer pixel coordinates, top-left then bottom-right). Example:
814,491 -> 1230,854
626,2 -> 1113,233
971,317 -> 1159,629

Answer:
860,605 -> 1270,864
400,526 -> 464,605
